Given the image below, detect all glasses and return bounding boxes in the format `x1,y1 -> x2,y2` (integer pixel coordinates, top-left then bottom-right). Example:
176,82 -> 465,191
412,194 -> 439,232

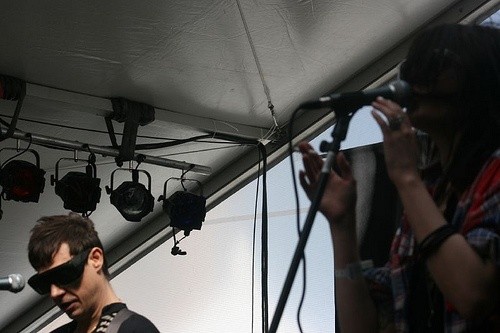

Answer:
27,247 -> 92,296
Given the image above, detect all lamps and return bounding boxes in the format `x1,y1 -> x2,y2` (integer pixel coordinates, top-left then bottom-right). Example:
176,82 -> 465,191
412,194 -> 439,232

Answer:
50,142 -> 103,218
105,154 -> 156,224
1,131 -> 47,205
157,164 -> 207,257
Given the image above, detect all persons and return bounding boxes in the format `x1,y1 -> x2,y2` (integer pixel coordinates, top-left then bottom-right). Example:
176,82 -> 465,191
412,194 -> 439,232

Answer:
26,212 -> 161,333
296,19 -> 500,333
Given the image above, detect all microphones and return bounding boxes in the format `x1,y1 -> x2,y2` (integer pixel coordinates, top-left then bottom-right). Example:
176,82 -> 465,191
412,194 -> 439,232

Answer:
0,273 -> 25,293
300,80 -> 413,111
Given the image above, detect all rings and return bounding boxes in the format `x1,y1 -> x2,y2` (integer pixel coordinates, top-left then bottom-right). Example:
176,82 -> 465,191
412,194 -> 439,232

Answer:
396,114 -> 405,124
